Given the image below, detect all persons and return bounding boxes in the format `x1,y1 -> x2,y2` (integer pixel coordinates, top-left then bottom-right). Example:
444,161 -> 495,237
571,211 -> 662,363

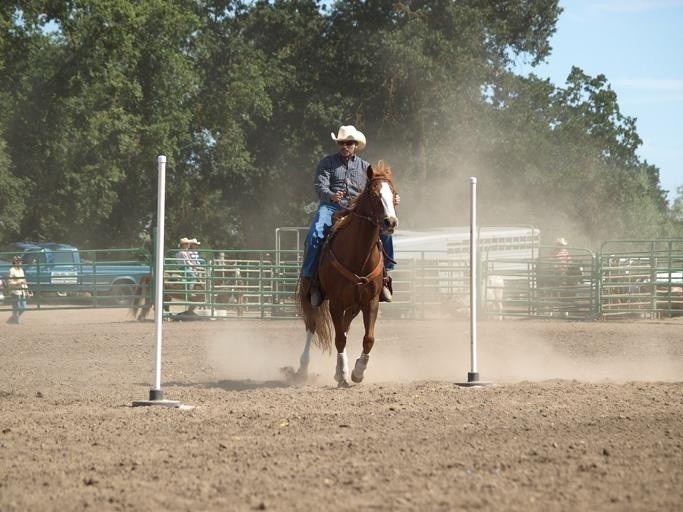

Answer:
174,234 -> 226,297
546,235 -> 572,290
297,125 -> 398,310
3,254 -> 29,325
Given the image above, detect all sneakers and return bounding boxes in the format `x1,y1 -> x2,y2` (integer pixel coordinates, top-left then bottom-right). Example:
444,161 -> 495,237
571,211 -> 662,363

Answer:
309,277 -> 323,306
382,283 -> 392,303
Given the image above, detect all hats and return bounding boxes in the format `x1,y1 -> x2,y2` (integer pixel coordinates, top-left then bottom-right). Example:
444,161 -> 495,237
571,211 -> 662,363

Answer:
177,237 -> 191,247
189,238 -> 201,245
330,125 -> 366,153
552,236 -> 568,245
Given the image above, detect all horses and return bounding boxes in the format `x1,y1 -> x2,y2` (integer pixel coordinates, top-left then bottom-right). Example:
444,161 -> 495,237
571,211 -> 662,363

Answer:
132,257 -> 245,322
540,259 -> 585,321
293,158 -> 399,388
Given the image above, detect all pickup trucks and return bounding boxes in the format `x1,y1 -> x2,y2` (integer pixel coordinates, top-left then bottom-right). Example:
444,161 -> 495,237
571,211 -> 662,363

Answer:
0,242 -> 153,308
